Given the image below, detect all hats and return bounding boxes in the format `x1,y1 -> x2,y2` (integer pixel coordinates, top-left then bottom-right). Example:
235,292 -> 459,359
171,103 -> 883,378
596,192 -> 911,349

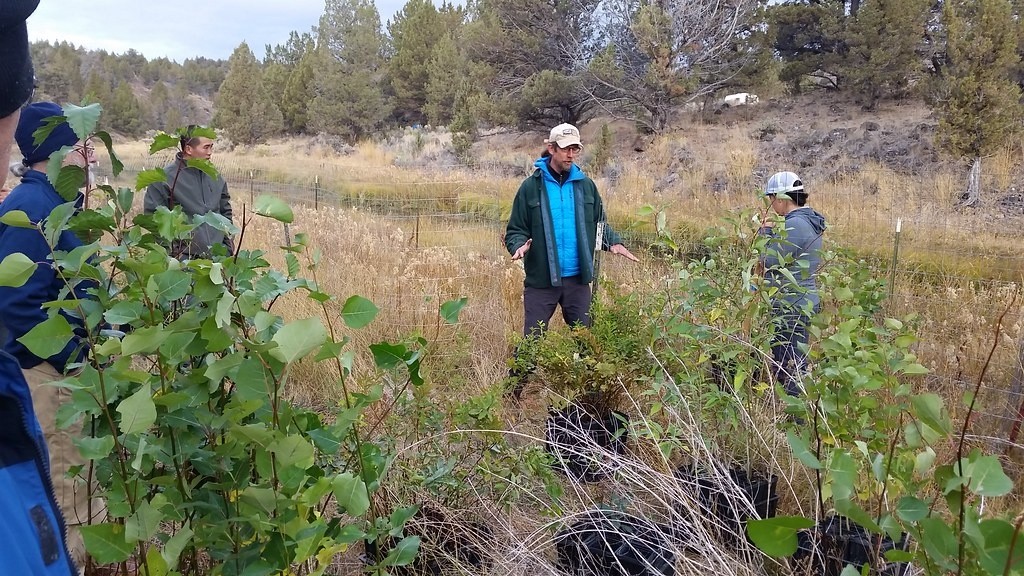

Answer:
543,123 -> 584,149
14,102 -> 80,168
0,0 -> 45,120
758,171 -> 804,199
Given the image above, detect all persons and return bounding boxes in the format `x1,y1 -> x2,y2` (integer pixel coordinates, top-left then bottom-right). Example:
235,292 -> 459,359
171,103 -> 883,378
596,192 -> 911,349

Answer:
502,123 -> 640,402
0,101 -> 112,576
144,125 -> 235,367
759,172 -> 825,424
0,0 -> 79,198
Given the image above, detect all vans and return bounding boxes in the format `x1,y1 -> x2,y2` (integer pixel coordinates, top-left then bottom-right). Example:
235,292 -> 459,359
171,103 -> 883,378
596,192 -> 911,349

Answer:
724,92 -> 758,106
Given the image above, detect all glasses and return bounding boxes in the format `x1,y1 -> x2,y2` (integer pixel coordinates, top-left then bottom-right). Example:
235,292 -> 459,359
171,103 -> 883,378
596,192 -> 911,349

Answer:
562,146 -> 581,154
74,144 -> 96,156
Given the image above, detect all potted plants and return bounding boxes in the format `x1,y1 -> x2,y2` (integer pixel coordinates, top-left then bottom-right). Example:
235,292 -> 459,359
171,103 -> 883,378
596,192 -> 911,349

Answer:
334,187 -> 1024,576
